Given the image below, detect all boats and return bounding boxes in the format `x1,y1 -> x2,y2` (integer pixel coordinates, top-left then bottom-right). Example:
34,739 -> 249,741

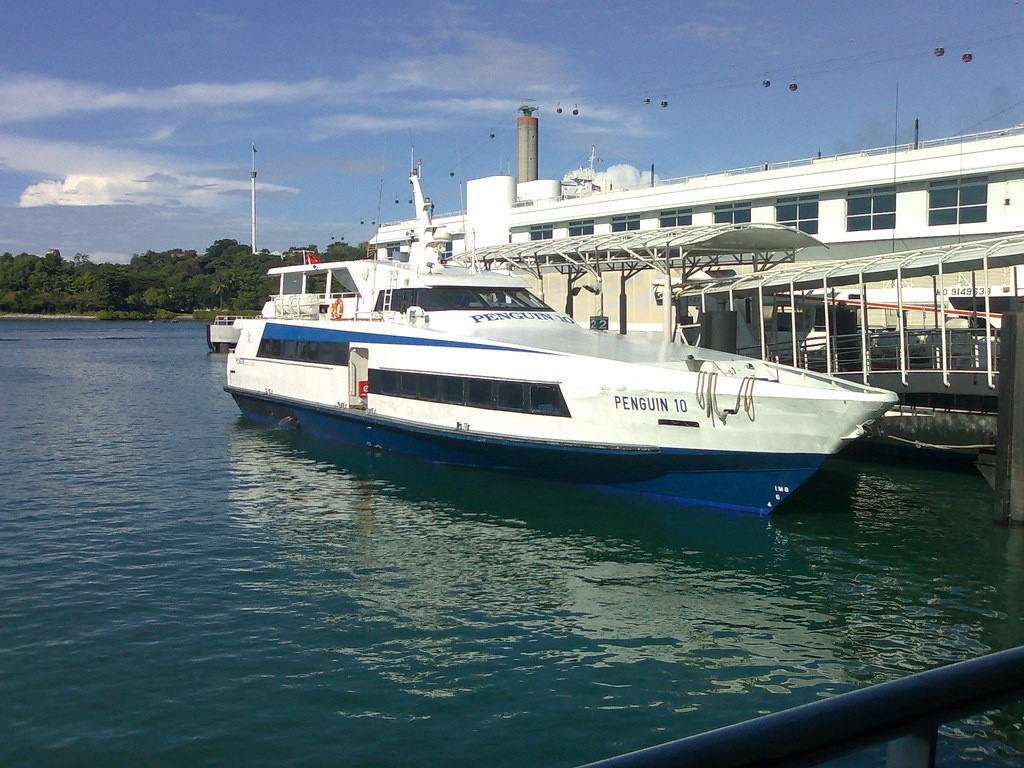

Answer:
222,223 -> 899,516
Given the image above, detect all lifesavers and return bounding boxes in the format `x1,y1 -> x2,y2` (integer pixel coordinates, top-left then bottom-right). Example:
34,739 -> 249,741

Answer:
309,254 -> 320,264
333,299 -> 343,319
